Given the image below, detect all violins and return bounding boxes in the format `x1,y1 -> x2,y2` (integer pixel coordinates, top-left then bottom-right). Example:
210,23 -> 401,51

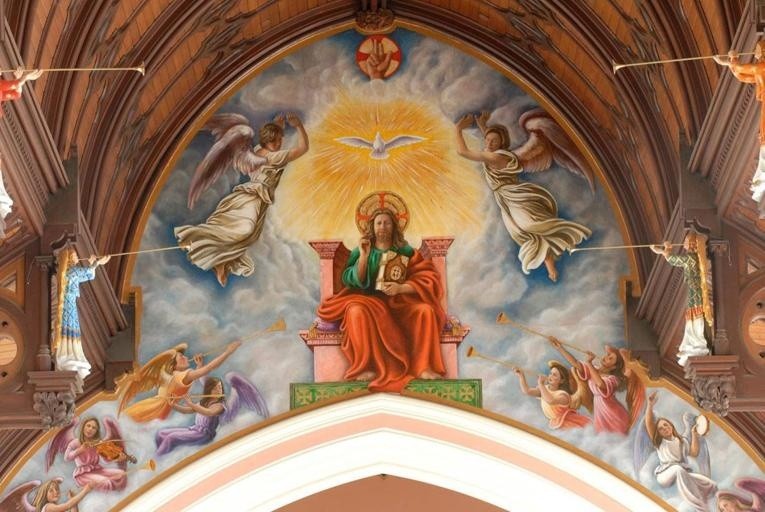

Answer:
87,438 -> 138,464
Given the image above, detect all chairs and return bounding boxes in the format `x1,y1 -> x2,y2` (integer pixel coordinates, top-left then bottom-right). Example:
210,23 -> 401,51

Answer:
296,230 -> 473,380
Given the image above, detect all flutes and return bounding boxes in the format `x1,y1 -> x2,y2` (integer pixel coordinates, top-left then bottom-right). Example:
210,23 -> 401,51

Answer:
153,393 -> 226,400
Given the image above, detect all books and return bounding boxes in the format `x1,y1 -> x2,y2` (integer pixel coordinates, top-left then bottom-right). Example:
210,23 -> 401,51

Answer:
375,250 -> 410,294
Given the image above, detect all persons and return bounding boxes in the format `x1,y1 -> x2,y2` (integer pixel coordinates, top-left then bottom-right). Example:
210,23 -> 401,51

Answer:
126,340 -> 241,420
454,109 -> 558,282
58,248 -> 111,376
704,491 -> 758,512
154,376 -> 224,459
34,476 -> 94,511
649,230 -> 707,349
713,37 -> 765,200
340,208 -> 445,382
211,110 -> 310,288
644,389 -> 717,509
513,363 -> 588,430
65,417 -> 126,491
550,337 -> 630,432
0,66 -> 43,218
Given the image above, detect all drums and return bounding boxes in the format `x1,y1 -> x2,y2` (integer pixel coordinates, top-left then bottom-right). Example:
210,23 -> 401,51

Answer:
695,413 -> 710,436
